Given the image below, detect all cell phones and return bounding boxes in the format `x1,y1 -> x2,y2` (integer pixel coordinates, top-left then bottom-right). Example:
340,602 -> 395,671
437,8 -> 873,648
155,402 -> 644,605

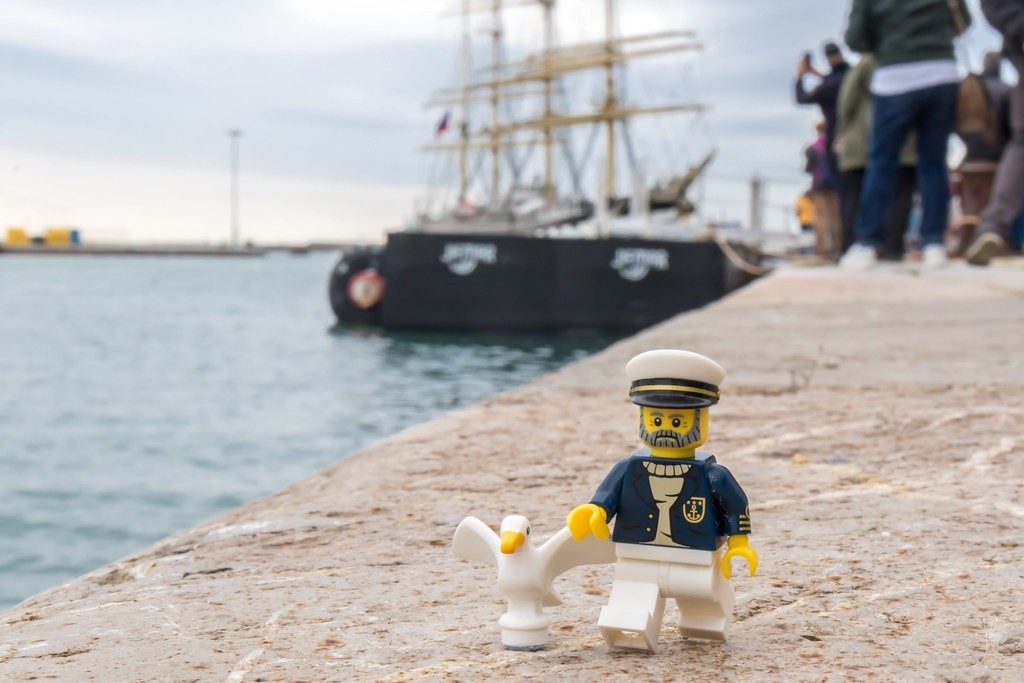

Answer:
805,54 -> 810,71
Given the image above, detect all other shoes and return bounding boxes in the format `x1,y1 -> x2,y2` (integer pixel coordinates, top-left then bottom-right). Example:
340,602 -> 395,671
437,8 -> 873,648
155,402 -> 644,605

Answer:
922,245 -> 946,264
842,246 -> 877,266
969,233 -> 1004,265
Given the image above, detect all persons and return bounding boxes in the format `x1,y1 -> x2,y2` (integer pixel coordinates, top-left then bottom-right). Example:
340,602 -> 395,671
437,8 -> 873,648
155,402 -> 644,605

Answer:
567,349 -> 760,654
793,0 -> 1024,270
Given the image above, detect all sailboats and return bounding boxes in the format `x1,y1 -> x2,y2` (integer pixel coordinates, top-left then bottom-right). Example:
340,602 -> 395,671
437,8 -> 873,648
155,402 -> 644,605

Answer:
383,0 -> 758,330
